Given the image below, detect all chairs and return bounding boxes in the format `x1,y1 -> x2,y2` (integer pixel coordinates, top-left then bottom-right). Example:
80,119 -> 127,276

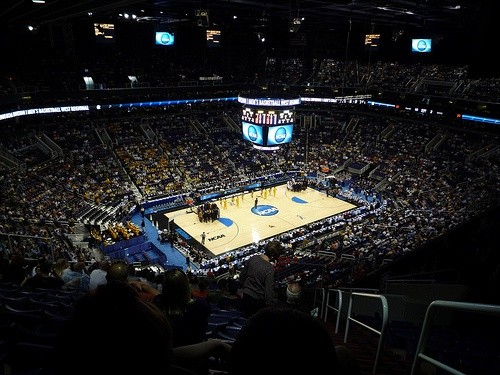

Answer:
0,61 -> 500,375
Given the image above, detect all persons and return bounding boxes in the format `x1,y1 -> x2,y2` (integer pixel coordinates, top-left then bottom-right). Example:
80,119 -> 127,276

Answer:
0,57 -> 500,375
238,241 -> 283,317
